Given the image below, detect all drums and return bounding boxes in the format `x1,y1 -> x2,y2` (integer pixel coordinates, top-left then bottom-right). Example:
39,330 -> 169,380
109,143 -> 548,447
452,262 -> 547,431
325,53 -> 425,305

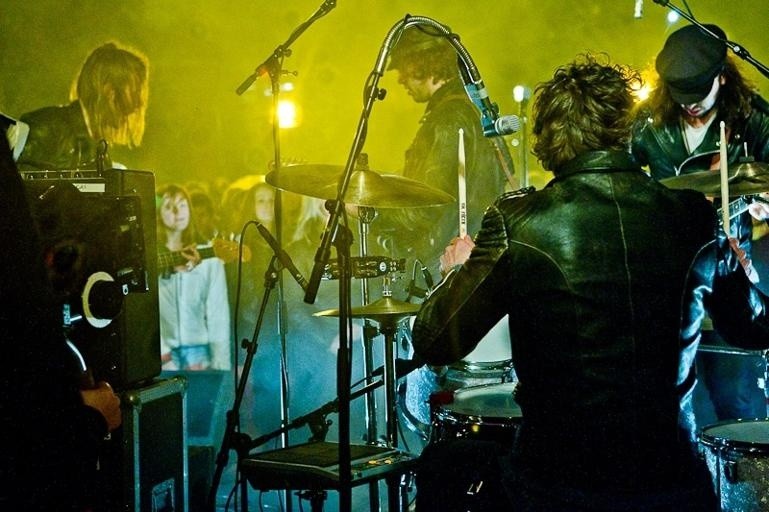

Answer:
425,386 -> 524,509
696,417 -> 769,512
394,300 -> 515,439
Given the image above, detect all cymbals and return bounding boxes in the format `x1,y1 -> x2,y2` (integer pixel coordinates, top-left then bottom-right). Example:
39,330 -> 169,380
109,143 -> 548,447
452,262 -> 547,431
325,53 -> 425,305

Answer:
265,164 -> 456,209
658,161 -> 769,198
312,297 -> 424,318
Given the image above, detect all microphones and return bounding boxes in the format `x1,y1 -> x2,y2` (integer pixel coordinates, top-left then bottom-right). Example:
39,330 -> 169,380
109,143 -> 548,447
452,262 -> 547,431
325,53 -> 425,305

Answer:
633,0 -> 642,20
97,144 -> 103,171
253,220 -> 309,292
481,114 -> 521,137
422,265 -> 433,290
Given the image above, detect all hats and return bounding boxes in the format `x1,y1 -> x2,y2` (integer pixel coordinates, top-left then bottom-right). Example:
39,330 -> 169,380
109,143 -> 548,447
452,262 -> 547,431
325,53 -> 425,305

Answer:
656,24 -> 727,104
386,26 -> 447,70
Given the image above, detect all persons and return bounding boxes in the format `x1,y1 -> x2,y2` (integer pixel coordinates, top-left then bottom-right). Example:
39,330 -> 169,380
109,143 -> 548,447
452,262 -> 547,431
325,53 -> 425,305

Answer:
1,24 -> 769,512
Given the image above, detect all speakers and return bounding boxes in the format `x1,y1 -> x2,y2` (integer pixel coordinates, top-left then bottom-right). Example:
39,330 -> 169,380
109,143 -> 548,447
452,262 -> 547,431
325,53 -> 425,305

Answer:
18,167 -> 164,387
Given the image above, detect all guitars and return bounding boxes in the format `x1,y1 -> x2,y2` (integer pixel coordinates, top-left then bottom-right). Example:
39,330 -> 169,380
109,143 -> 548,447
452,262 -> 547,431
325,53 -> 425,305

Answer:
714,190 -> 769,229
156,229 -> 253,285
